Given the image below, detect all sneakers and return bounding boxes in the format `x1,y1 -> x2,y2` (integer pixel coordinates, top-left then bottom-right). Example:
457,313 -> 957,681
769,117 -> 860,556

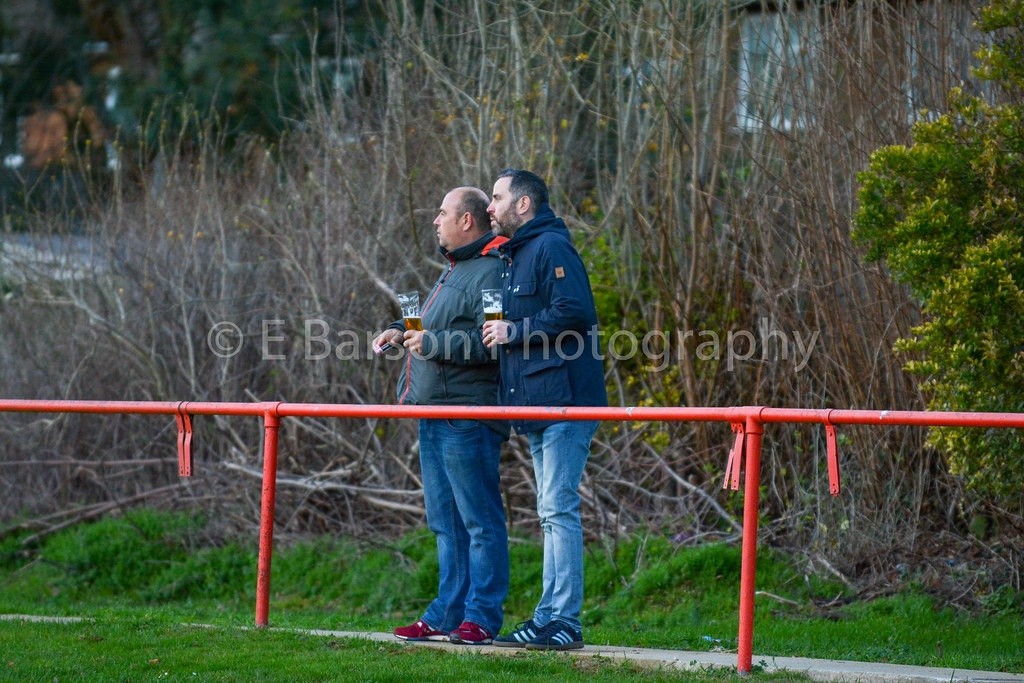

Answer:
492,618 -> 585,651
393,619 -> 450,642
449,621 -> 493,645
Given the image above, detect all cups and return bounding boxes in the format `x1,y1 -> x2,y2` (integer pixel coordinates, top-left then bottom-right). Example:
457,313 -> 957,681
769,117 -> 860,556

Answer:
482,289 -> 504,344
399,292 -> 422,331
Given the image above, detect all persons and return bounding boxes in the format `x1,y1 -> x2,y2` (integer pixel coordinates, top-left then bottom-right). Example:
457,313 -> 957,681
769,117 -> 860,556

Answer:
481,169 -> 609,651
373,186 -> 513,647
